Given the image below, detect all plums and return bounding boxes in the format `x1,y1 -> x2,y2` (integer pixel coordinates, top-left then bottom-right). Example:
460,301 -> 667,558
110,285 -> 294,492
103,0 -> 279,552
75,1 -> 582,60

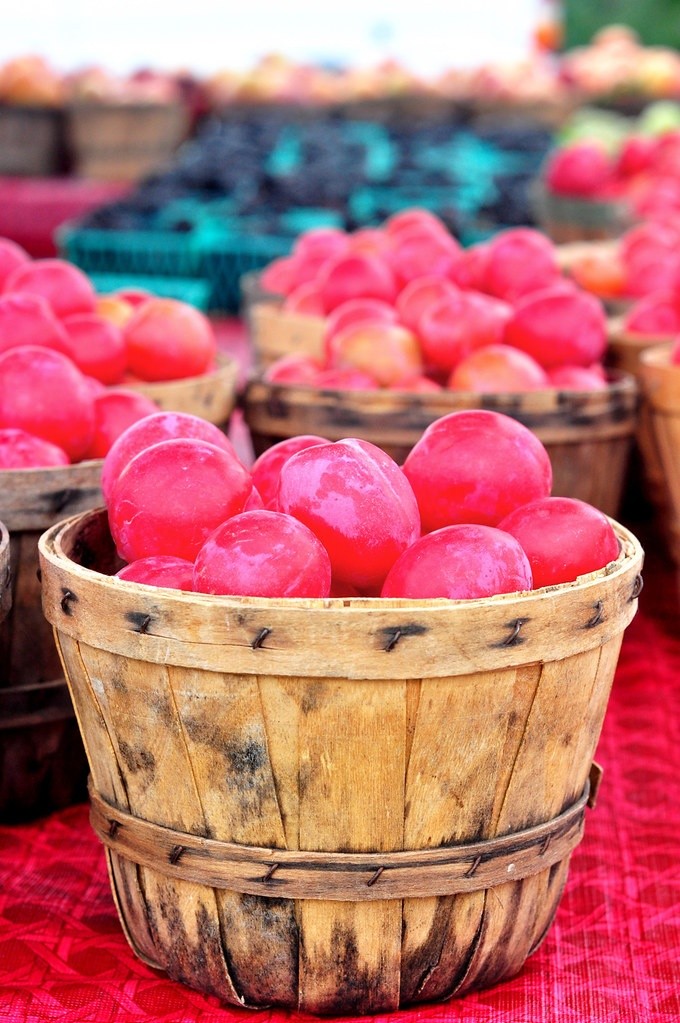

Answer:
101,408 -> 621,602
0,117 -> 680,469
